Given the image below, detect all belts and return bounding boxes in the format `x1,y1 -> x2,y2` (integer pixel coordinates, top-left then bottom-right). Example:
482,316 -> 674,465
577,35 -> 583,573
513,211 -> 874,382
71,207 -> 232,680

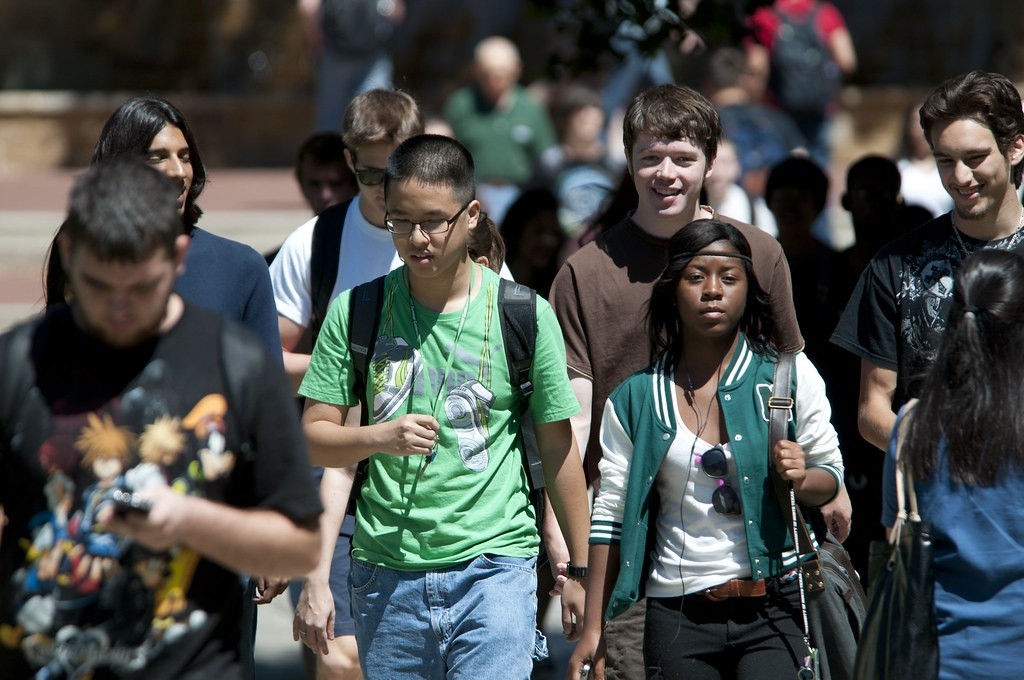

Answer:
697,565 -> 797,602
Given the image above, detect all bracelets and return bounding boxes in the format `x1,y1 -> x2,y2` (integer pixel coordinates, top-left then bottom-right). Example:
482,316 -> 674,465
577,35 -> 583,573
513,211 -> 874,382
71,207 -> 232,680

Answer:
567,561 -> 589,581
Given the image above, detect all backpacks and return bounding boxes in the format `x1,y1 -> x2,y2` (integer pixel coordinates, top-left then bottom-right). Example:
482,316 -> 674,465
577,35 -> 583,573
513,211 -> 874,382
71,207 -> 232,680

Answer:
770,2 -> 842,113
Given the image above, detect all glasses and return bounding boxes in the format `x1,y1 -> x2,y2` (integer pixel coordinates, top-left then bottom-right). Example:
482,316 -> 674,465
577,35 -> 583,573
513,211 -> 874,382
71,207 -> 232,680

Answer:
351,152 -> 387,186
384,205 -> 466,234
702,443 -> 741,517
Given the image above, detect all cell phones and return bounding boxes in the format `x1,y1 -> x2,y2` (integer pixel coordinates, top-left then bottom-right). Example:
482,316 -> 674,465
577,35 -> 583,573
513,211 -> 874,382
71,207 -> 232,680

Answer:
113,491 -> 152,521
580,662 -> 593,680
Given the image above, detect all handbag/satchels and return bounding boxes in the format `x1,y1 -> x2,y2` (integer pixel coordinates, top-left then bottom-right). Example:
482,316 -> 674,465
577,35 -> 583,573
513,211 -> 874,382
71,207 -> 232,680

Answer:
797,529 -> 869,680
852,398 -> 940,680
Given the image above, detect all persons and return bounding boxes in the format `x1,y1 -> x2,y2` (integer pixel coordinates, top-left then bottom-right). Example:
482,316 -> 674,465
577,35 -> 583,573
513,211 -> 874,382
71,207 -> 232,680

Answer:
811,152 -> 936,550
293,133 -> 590,679
830,70 -> 1024,453
298,1 -> 405,131
0,154 -> 326,679
439,37 -> 557,231
889,97 -> 957,218
30,95 -> 291,679
263,130 -> 359,267
529,86 -> 627,268
764,152 -> 869,591
594,0 -> 856,248
705,138 -> 777,239
566,220 -> 844,680
880,249 -> 1023,679
269,85 -> 517,679
549,83 -> 805,680
296,211 -> 571,658
